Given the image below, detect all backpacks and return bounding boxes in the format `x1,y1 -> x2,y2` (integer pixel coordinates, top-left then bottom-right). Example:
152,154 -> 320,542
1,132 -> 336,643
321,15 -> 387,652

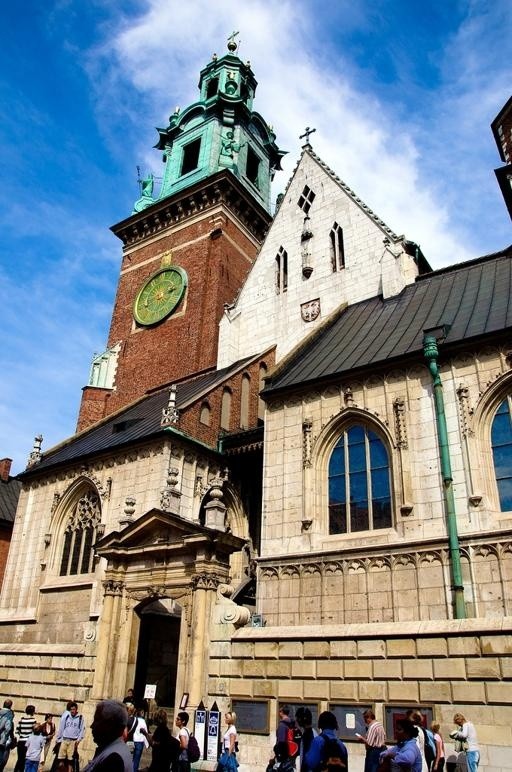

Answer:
184,727 -> 200,763
313,732 -> 347,771
279,718 -> 302,758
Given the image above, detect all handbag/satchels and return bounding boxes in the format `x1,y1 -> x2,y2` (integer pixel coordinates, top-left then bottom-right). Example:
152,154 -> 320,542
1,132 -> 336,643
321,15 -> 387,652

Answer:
425,744 -> 435,762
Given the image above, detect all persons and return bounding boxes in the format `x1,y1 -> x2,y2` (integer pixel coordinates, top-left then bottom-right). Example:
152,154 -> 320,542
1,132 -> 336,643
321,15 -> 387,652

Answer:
14,705 -> 55,772
55,702 -> 86,772
266,704 -> 348,772
83,699 -> 134,772
146,709 -> 171,771
122,688 -> 149,771
450,712 -> 480,772
355,709 -> 445,772
0,698 -> 14,772
175,712 -> 191,771
216,711 -> 237,772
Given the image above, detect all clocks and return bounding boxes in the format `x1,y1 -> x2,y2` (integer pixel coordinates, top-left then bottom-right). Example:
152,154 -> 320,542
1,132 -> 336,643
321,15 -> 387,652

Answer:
131,263 -> 188,329
